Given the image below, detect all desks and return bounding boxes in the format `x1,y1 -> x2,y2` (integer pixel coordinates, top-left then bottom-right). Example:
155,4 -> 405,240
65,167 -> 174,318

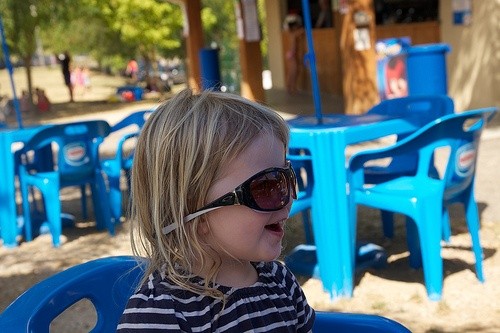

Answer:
285,113 -> 413,297
0,125 -> 56,247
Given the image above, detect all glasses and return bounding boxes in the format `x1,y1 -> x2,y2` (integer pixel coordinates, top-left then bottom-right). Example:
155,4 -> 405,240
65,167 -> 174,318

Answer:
151,159 -> 298,240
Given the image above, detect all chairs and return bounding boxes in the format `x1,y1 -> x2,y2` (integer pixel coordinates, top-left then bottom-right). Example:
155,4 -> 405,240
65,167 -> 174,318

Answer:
0,95 -> 497,333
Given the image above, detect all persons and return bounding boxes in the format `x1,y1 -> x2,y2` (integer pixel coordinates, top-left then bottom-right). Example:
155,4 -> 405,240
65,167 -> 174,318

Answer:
57,50 -> 141,105
116,92 -> 317,333
